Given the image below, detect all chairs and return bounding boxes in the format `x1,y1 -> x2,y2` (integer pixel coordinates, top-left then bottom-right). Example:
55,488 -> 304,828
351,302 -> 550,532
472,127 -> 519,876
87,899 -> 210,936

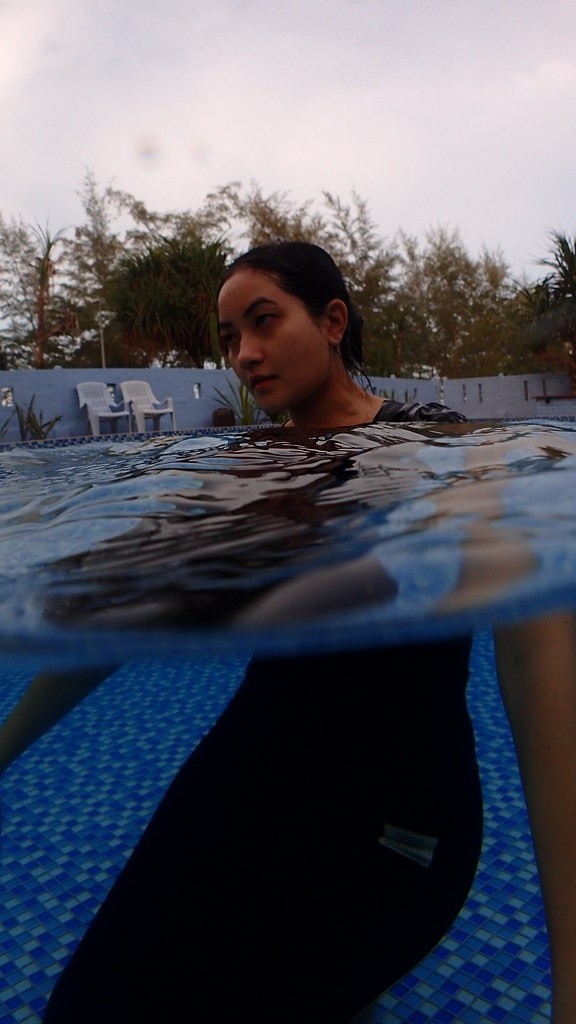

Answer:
75,381 -> 134,437
119,380 -> 177,433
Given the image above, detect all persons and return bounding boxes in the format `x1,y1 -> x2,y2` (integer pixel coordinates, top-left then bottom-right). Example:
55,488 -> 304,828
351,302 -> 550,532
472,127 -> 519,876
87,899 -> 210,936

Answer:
216,242 -> 472,428
